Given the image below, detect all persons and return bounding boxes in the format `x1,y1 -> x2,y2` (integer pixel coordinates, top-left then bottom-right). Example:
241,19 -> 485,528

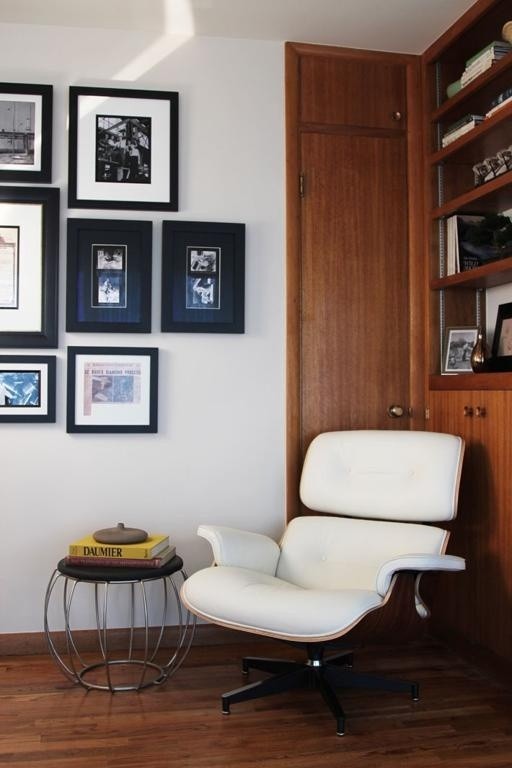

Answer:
105,126 -> 150,182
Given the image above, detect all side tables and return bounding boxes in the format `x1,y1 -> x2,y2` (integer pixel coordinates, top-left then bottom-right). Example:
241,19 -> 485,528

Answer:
42,555 -> 195,689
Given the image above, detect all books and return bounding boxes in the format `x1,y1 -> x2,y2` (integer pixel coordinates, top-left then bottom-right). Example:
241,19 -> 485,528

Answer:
440,95 -> 511,149
459,38 -> 511,91
443,213 -> 503,279
69,531 -> 170,560
65,544 -> 177,569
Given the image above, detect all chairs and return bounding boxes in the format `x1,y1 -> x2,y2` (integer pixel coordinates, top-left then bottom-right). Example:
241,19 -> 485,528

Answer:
180,429 -> 466,735
